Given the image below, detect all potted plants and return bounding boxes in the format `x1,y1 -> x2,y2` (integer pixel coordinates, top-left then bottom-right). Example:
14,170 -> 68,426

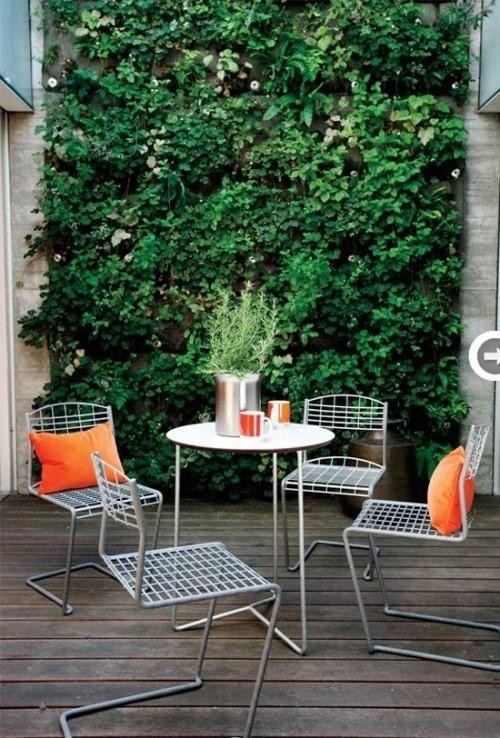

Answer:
194,280 -> 280,437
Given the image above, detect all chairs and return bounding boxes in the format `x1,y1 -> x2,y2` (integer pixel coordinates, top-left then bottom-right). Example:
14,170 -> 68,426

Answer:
342,425 -> 500,672
25,402 -> 163,615
281,394 -> 388,581
59,451 -> 282,738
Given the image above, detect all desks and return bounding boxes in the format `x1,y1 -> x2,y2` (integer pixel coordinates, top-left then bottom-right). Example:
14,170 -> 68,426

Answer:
166,422 -> 335,656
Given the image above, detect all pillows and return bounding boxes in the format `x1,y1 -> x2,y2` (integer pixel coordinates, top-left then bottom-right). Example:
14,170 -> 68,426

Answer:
29,422 -> 127,495
427,445 -> 476,536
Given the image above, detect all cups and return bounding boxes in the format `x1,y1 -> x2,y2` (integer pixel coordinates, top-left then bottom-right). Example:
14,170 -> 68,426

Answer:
240,411 -> 268,437
267,400 -> 291,426
